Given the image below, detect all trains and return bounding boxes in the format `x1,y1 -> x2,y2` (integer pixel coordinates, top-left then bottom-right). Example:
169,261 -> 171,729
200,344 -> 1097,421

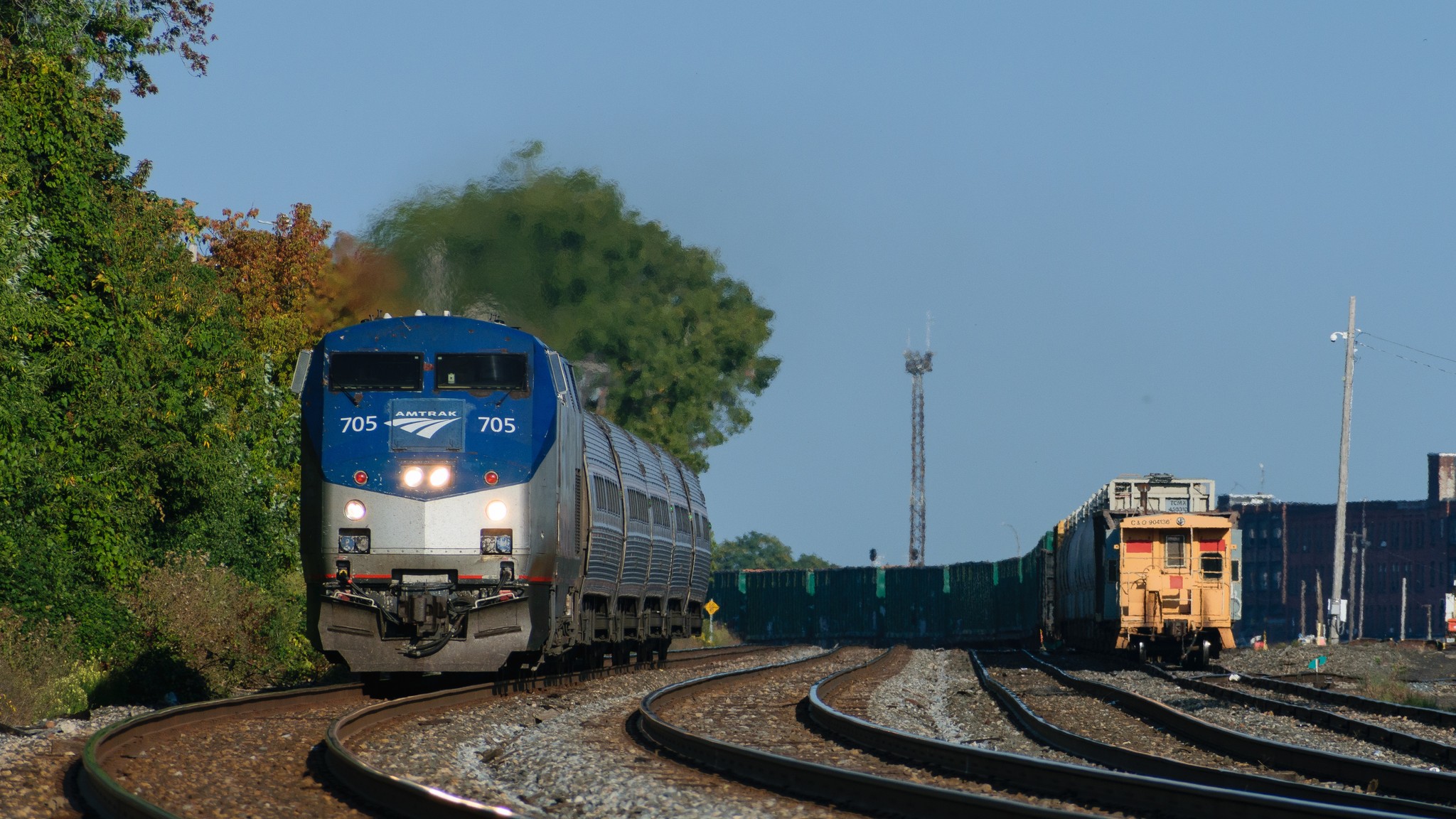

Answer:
702,472 -> 1237,671
291,309 -> 713,699
